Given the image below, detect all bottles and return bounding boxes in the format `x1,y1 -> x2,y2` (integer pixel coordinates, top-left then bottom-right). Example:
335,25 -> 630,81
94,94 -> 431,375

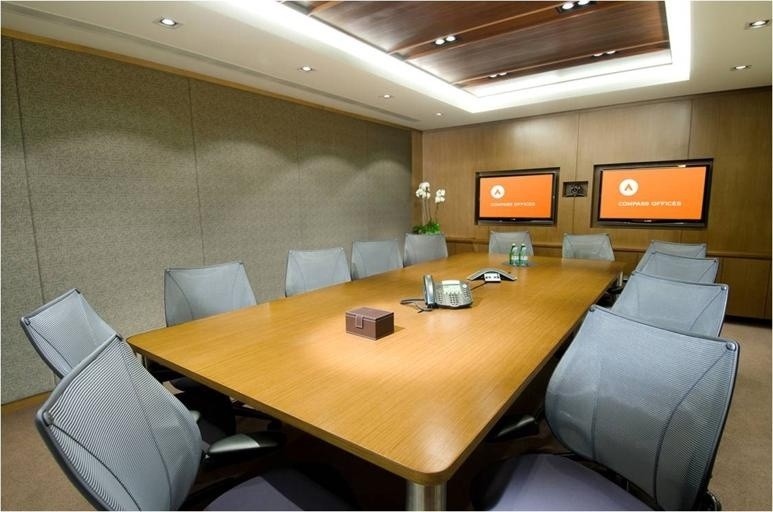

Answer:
508,243 -> 528,267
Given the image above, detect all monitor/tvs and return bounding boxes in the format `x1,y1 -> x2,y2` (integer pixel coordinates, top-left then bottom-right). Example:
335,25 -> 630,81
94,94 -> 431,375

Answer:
475,167 -> 560,226
590,158 -> 714,229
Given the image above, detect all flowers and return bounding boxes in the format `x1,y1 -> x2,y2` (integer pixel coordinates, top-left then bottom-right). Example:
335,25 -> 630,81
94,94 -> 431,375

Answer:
412,181 -> 445,234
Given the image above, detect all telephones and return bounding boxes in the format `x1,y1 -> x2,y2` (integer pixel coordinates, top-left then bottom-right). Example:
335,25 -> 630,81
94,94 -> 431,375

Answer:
423,275 -> 473,309
468,267 -> 518,283
